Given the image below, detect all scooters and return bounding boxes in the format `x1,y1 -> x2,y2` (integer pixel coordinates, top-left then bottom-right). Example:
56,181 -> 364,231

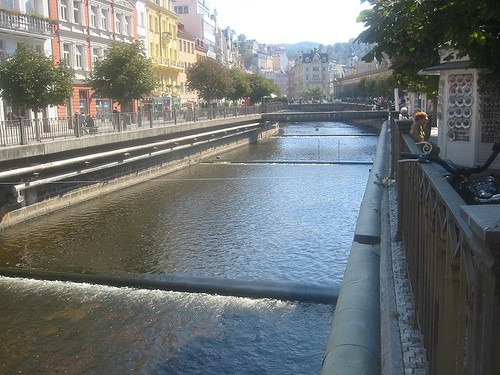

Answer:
396,141 -> 500,206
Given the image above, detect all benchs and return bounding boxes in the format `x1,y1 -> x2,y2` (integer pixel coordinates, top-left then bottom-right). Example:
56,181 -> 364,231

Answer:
73,126 -> 98,136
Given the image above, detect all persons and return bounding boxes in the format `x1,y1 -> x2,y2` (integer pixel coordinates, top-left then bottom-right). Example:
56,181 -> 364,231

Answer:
288,95 -> 342,103
183,106 -> 188,112
398,97 -> 430,140
194,96 -> 254,120
347,95 -> 392,111
78,107 -> 87,137
113,106 -> 118,113
84,114 -> 94,135
